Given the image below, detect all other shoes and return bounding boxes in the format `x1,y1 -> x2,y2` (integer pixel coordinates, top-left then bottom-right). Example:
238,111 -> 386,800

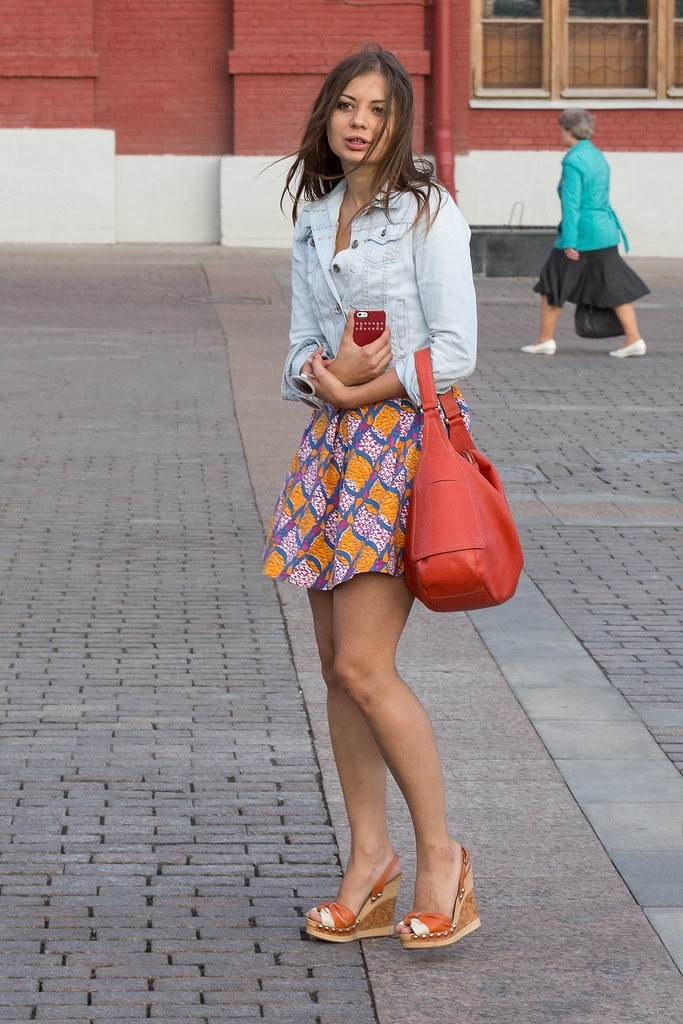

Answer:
521,339 -> 557,354
609,338 -> 646,357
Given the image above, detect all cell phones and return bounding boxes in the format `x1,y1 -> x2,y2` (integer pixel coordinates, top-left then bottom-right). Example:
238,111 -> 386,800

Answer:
353,309 -> 386,347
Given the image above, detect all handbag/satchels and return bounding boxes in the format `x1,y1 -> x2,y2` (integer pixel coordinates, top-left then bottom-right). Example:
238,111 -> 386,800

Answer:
405,347 -> 524,613
574,300 -> 625,338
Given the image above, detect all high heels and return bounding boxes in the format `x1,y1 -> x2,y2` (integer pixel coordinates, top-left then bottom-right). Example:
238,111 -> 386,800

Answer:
305,853 -> 401,942
398,846 -> 481,948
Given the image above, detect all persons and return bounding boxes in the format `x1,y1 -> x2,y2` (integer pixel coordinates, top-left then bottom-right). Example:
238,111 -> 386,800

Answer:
521,108 -> 652,359
260,46 -> 480,949
